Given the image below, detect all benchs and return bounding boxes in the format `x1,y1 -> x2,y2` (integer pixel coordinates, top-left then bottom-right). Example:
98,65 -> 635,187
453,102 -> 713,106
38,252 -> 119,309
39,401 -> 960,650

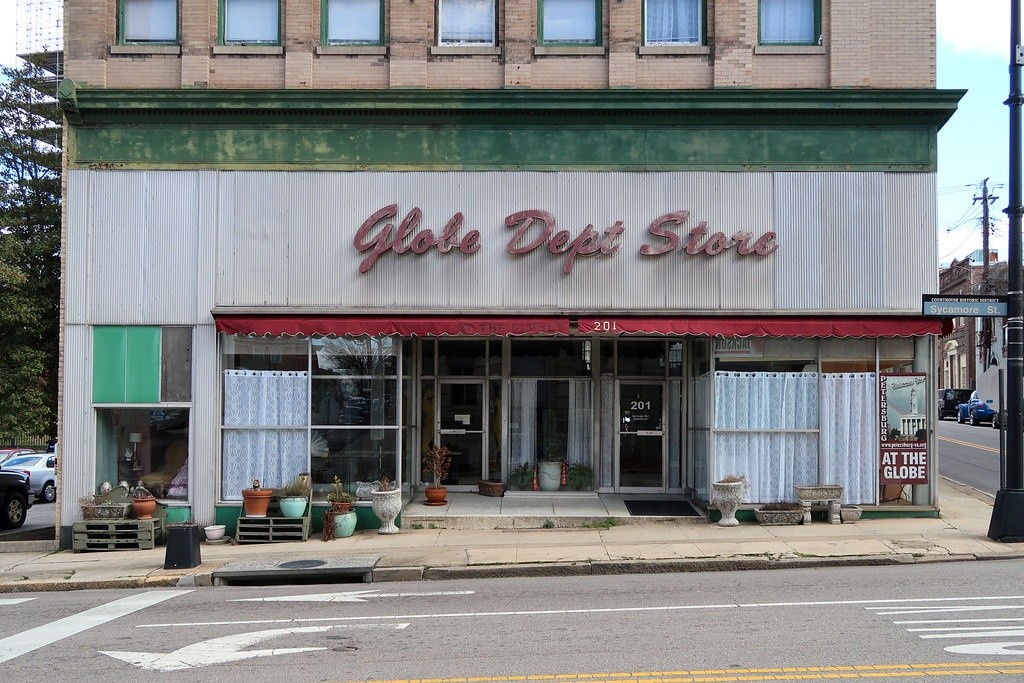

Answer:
71,486 -> 167,553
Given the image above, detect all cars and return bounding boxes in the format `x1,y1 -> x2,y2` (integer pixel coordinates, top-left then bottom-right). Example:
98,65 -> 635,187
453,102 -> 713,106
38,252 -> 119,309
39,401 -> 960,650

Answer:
957,389 -> 996,426
0,453 -> 57,504
46,438 -> 57,454
0,446 -> 39,470
0,468 -> 35,531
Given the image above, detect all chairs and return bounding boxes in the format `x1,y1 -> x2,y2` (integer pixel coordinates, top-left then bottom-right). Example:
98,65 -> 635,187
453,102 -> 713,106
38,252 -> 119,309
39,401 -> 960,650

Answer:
141,440 -> 187,499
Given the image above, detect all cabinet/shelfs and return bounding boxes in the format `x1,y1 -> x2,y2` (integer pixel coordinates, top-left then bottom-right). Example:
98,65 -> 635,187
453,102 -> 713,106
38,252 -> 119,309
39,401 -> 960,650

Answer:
118,459 -> 141,494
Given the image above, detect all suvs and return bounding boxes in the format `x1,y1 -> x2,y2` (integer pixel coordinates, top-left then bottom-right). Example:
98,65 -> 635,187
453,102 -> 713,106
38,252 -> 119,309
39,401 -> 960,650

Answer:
938,388 -> 973,421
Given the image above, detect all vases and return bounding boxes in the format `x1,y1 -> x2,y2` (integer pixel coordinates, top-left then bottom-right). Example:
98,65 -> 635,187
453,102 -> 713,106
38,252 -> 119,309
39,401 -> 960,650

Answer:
203,524 -> 226,540
241,489 -> 273,517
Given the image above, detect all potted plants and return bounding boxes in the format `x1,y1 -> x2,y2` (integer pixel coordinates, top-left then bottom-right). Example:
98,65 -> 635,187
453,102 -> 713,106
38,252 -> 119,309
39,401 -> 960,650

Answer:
371,476 -> 402,534
755,478 -> 863,526
75,486 -> 156,521
163,517 -> 202,569
280,471 -> 313,518
711,472 -> 751,527
422,445 -> 452,503
322,474 -> 358,541
504,448 -> 594,492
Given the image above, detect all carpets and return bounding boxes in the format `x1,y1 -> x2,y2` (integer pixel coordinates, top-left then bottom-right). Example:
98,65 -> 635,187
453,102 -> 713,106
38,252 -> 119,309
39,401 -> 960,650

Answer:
623,499 -> 701,516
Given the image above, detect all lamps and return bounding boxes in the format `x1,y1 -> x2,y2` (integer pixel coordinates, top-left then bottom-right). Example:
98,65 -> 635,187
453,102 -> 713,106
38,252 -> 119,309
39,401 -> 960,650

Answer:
129,432 -> 142,470
